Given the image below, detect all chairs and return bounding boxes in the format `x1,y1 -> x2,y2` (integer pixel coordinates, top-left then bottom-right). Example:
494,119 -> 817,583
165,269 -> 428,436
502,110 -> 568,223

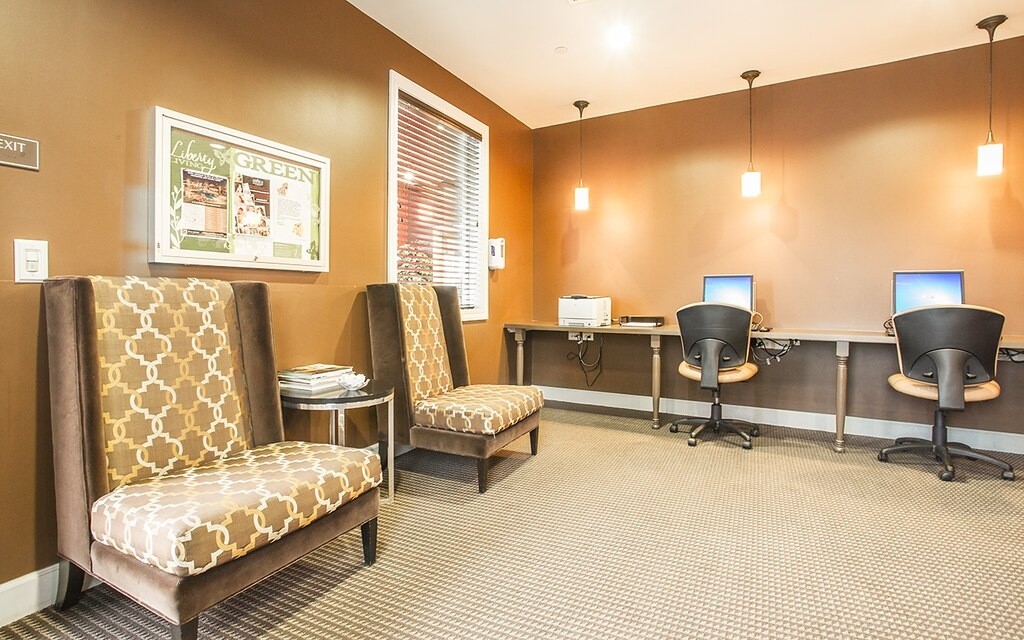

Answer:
43,276 -> 383,640
670,302 -> 760,449
878,305 -> 1016,484
366,284 -> 544,494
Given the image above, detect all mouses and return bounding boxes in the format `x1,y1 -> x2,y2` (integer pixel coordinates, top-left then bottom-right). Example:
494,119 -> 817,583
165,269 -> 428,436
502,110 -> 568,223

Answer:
758,326 -> 770,332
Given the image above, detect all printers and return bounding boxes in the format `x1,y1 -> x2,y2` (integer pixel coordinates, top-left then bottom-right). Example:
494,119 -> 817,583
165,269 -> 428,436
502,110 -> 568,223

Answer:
557,296 -> 612,328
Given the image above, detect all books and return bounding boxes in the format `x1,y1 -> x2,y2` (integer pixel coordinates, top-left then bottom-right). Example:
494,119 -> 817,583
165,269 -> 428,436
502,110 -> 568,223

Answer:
275,363 -> 354,391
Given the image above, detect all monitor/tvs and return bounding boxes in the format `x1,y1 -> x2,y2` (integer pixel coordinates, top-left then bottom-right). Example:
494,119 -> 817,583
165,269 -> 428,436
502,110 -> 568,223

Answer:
702,274 -> 754,316
890,270 -> 964,320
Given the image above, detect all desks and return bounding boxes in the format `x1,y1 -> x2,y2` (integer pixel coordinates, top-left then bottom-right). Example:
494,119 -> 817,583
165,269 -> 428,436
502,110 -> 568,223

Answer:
504,322 -> 1023,453
281,380 -> 395,504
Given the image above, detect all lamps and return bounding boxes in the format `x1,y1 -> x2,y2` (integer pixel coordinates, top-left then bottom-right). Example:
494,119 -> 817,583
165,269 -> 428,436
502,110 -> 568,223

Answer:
975,15 -> 1009,177
740,69 -> 761,198
573,101 -> 588,212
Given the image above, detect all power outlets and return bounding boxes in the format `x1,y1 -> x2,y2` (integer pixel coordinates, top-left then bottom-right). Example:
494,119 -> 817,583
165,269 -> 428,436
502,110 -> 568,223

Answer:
568,332 -> 581,340
582,332 -> 594,341
755,339 -> 768,348
